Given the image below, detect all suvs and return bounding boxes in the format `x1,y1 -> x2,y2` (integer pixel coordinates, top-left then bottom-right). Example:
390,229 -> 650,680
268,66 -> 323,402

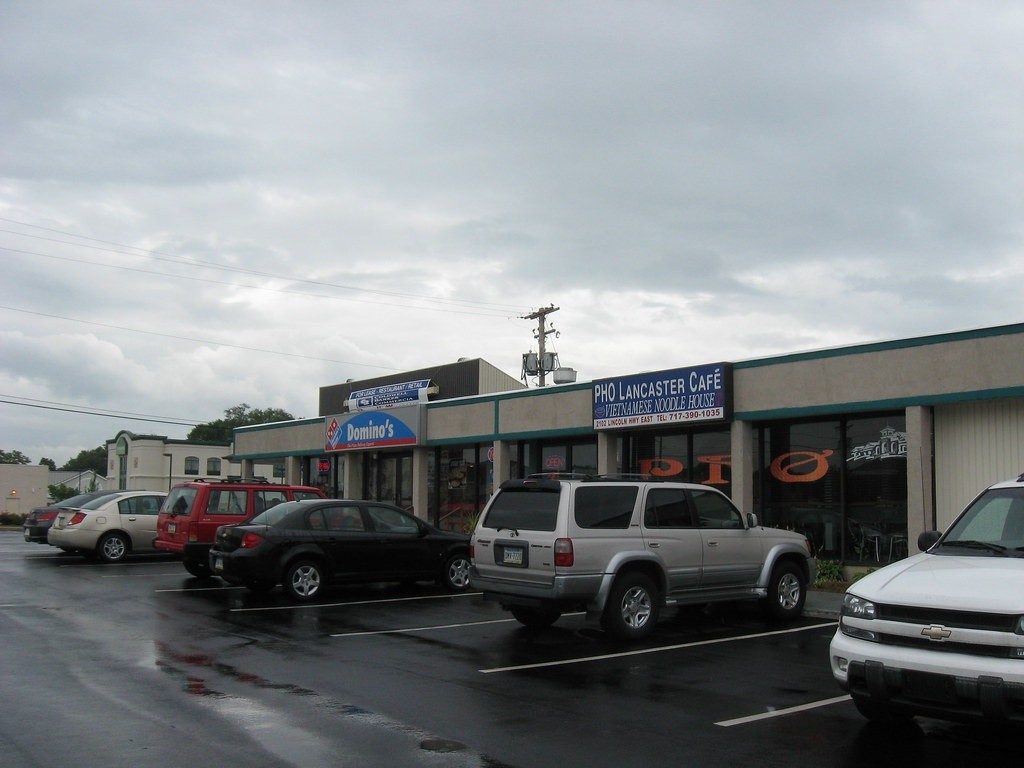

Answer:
824,471 -> 1023,733
466,470 -> 817,646
155,472 -> 378,583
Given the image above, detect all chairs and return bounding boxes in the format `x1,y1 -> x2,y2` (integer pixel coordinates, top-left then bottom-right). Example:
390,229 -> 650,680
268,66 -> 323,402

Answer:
255,497 -> 281,514
139,500 -> 154,515
769,511 -> 908,565
338,515 -> 358,532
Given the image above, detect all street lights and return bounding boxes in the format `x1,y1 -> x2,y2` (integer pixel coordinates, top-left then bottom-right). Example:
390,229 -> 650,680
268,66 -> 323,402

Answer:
162,453 -> 173,493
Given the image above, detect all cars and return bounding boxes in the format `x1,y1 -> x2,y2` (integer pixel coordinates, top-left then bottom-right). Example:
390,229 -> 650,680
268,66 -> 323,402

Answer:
46,488 -> 175,565
208,499 -> 473,600
24,491 -> 140,544
